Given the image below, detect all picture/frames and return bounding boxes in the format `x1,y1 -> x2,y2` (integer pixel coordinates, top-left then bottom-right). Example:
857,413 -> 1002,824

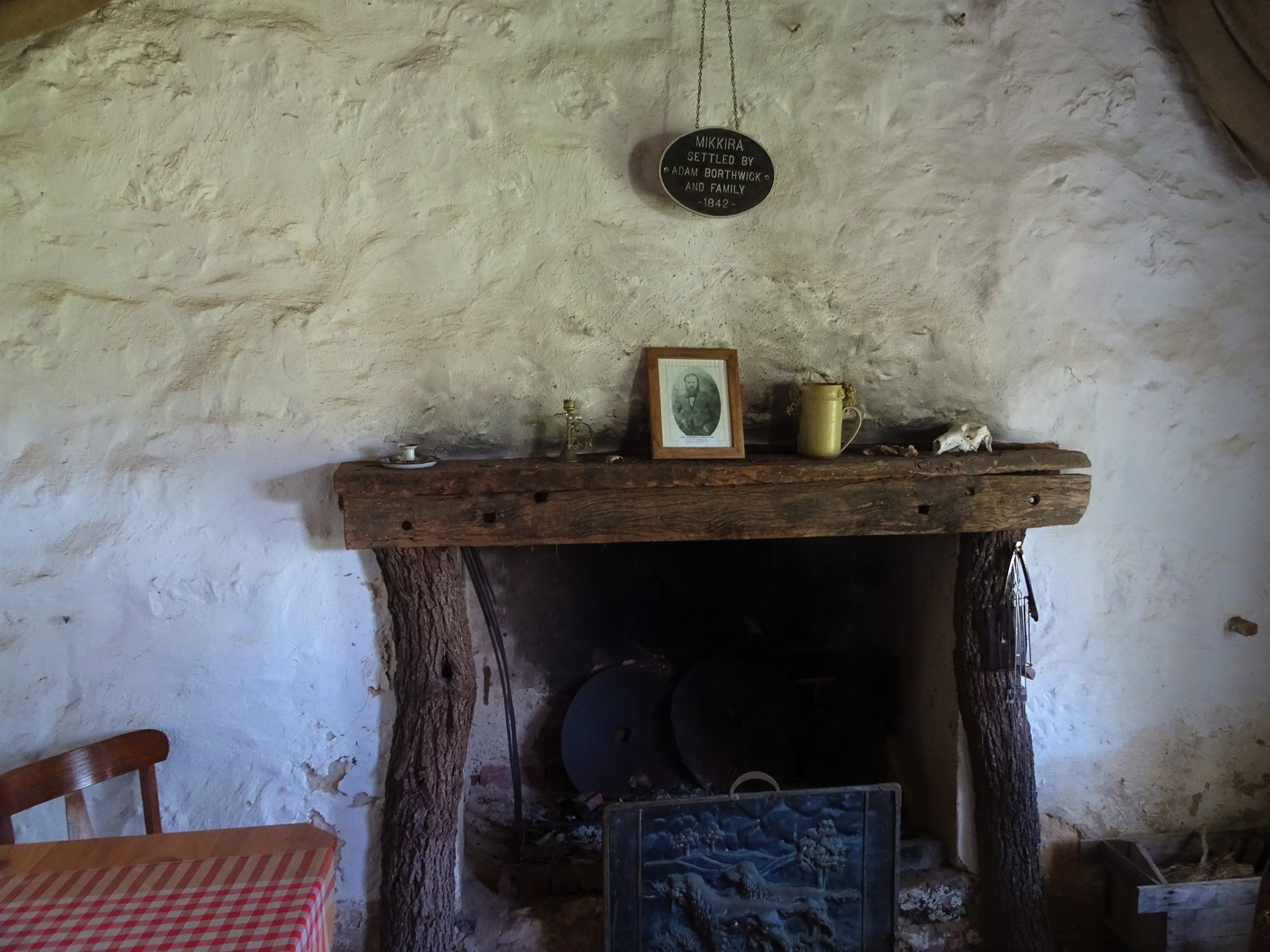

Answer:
646,347 -> 746,459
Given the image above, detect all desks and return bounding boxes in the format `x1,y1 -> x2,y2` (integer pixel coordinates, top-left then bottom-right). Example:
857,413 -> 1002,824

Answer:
0,823 -> 345,952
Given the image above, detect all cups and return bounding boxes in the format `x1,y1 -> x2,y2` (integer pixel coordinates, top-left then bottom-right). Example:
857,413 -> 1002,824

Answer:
399,444 -> 419,461
797,381 -> 862,460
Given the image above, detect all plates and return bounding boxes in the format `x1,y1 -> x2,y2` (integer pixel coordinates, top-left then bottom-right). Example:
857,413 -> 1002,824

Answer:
376,454 -> 438,469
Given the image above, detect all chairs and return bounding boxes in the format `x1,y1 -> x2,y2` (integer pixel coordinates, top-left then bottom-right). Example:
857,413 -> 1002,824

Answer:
0,729 -> 169,844
